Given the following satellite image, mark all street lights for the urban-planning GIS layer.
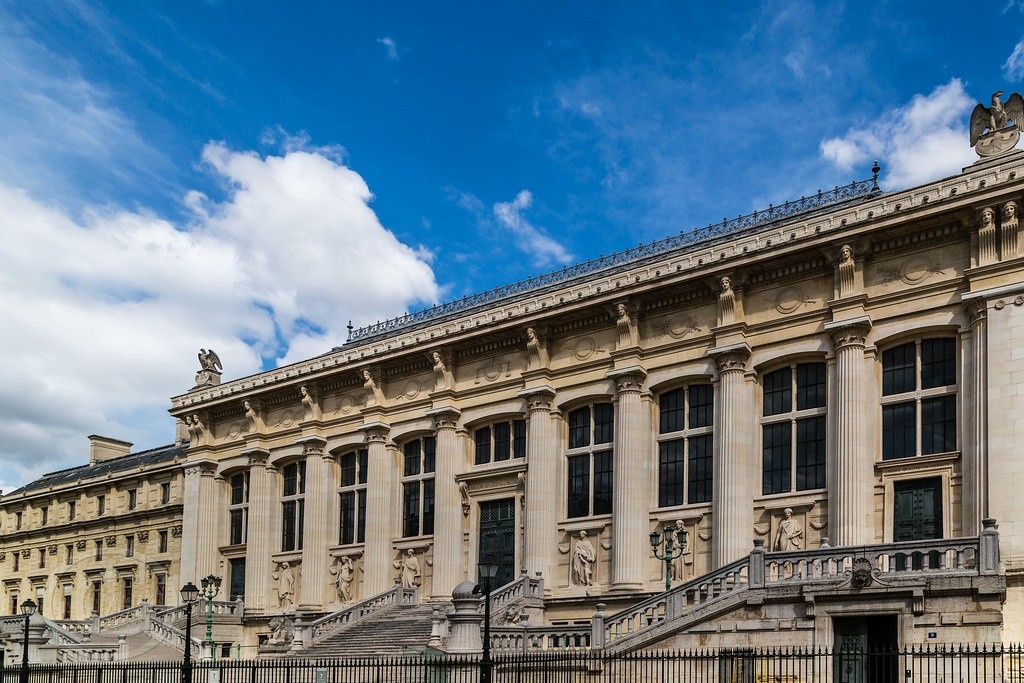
[179,581,199,683]
[648,525,688,592]
[19,599,38,683]
[471,553,500,683]
[201,574,222,641]
